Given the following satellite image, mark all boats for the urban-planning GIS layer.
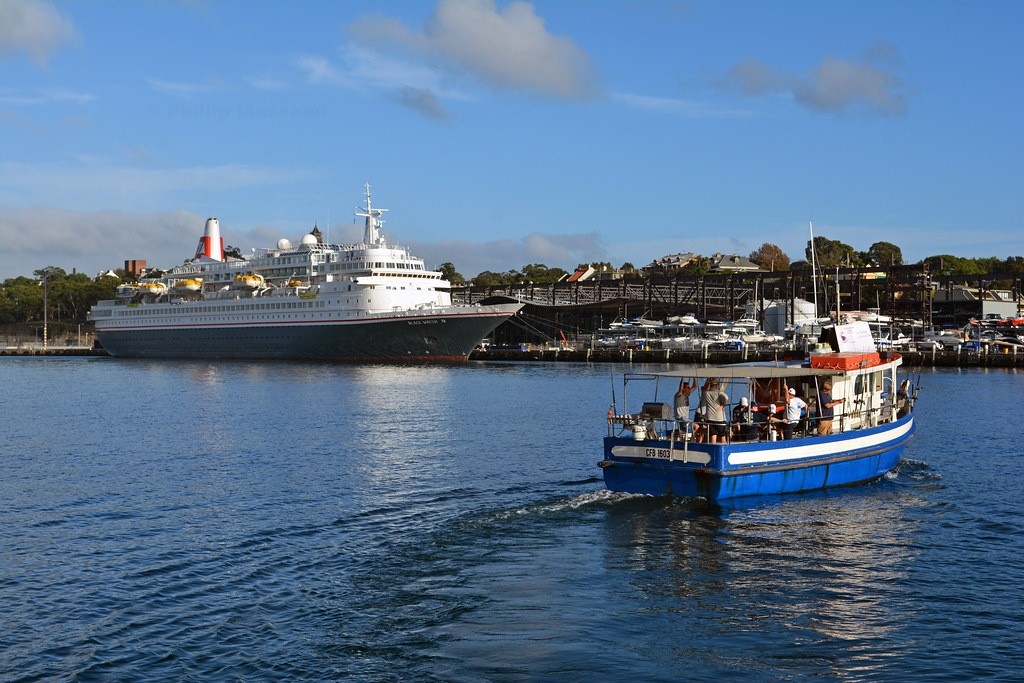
[600,318,919,494]
[519,308,1024,358]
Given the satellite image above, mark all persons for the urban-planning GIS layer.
[816,380,846,436]
[885,382,908,423]
[694,377,729,443]
[740,401,791,442]
[733,397,748,423]
[783,385,809,440]
[673,377,698,434]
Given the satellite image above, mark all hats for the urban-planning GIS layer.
[768,404,776,413]
[788,388,796,395]
[740,397,749,407]
[751,401,758,406]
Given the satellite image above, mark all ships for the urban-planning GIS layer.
[86,184,525,370]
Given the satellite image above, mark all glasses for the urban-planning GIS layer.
[686,387,690,389]
